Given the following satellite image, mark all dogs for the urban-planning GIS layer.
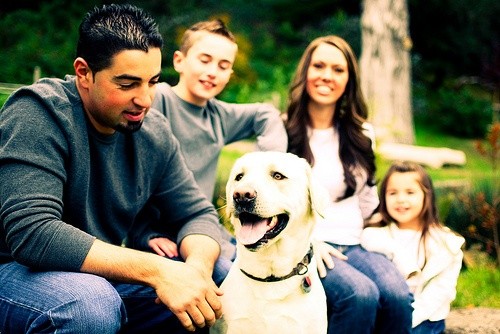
[207,151,328,334]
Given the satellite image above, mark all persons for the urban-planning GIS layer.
[274,34,415,334]
[123,16,288,262]
[360,159,465,334]
[0,3,235,334]
